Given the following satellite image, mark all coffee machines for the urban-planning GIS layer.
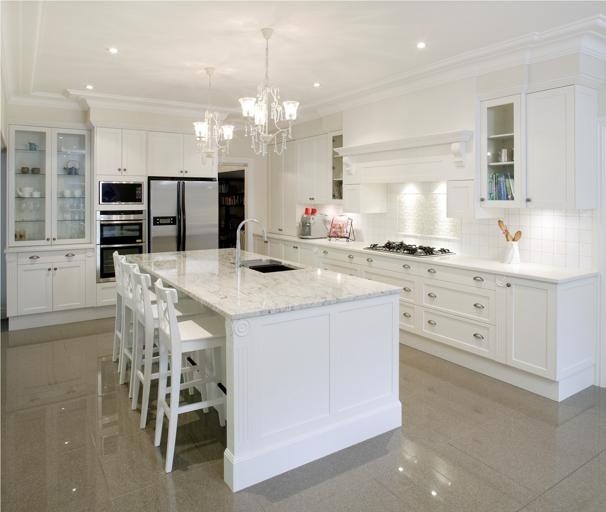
[298,206,329,238]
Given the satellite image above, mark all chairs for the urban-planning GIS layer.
[113,250,226,473]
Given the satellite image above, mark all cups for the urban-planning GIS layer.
[503,240,520,264]
[501,148,508,162]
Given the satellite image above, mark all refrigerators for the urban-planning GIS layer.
[148,175,219,255]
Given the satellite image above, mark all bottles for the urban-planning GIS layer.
[15,136,85,240]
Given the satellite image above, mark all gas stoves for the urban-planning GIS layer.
[364,240,455,258]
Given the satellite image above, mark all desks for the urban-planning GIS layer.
[125,247,405,494]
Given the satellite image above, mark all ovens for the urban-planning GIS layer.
[95,173,149,284]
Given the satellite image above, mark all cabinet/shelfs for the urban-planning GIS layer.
[267,142,297,234]
[297,135,328,204]
[96,127,147,176]
[253,236,282,261]
[146,130,213,177]
[328,130,343,203]
[7,124,91,247]
[16,259,85,315]
[473,88,525,209]
[525,85,598,211]
[284,240,319,270]
[505,277,599,382]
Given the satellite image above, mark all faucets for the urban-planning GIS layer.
[236,218,268,267]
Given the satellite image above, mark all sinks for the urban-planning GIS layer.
[249,263,305,274]
[230,258,282,267]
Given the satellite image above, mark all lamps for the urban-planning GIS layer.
[238,27,299,158]
[191,66,234,165]
